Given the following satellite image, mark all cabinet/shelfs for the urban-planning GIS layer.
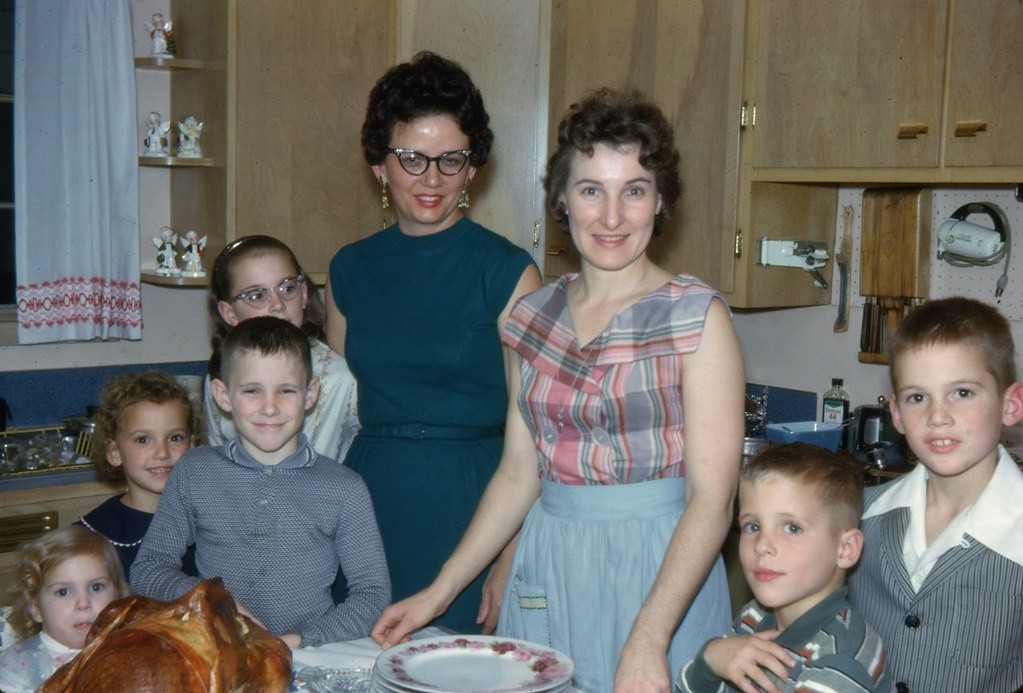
[0,479,131,608]
[542,0,1023,310]
[133,0,398,287]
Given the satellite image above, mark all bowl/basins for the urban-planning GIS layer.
[765,422,843,455]
[740,436,779,471]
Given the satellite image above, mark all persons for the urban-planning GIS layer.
[178,116,204,154]
[145,112,168,153]
[368,80,745,693]
[204,234,363,464]
[325,50,546,637]
[734,296,1023,693]
[150,226,179,269]
[151,13,169,54]
[130,315,392,650]
[180,230,207,273]
[676,440,892,693]
[72,368,202,584]
[0,524,124,693]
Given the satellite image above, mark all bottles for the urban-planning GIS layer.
[822,378,850,451]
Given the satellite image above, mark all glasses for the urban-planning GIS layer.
[226,267,304,309]
[384,146,472,176]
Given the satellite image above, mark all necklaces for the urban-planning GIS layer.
[554,258,653,431]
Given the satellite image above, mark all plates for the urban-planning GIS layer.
[369,634,576,693]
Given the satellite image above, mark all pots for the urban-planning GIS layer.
[62,404,98,436]
